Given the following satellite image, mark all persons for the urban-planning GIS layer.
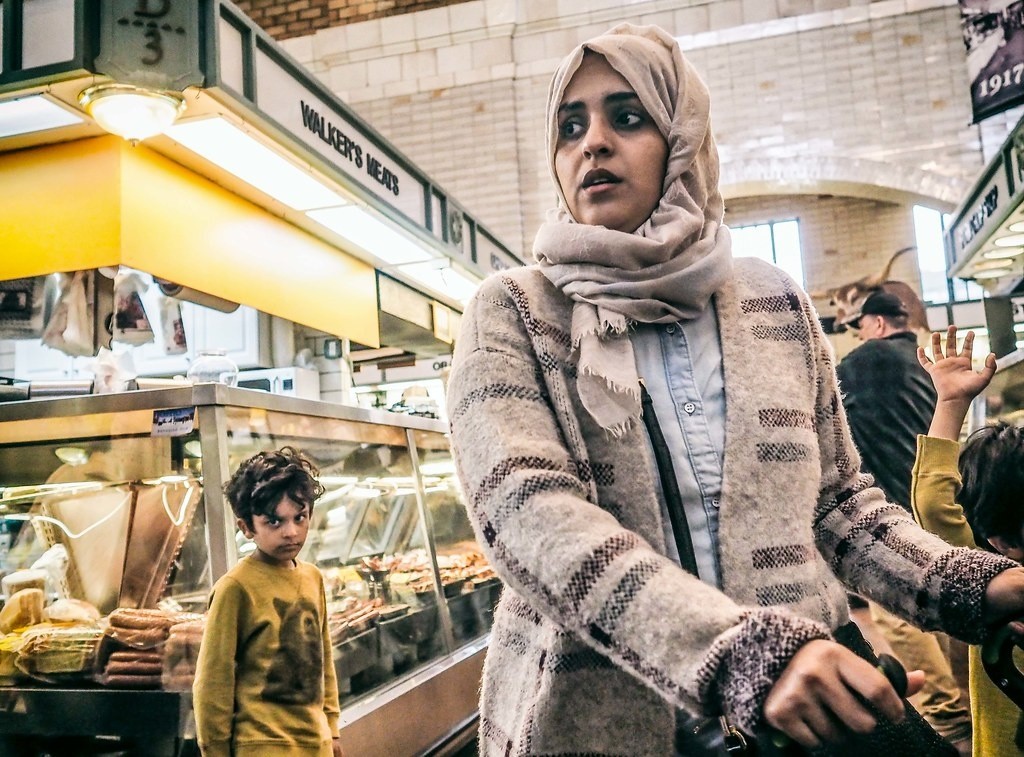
[823,291,972,757]
[192,448,342,757]
[911,326,1024,755]
[447,23,1024,757]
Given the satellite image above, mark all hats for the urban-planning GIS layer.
[840,292,910,329]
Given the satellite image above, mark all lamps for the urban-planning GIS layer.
[79,84,186,147]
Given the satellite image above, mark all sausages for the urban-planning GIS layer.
[327,597,381,638]
[101,607,208,690]
